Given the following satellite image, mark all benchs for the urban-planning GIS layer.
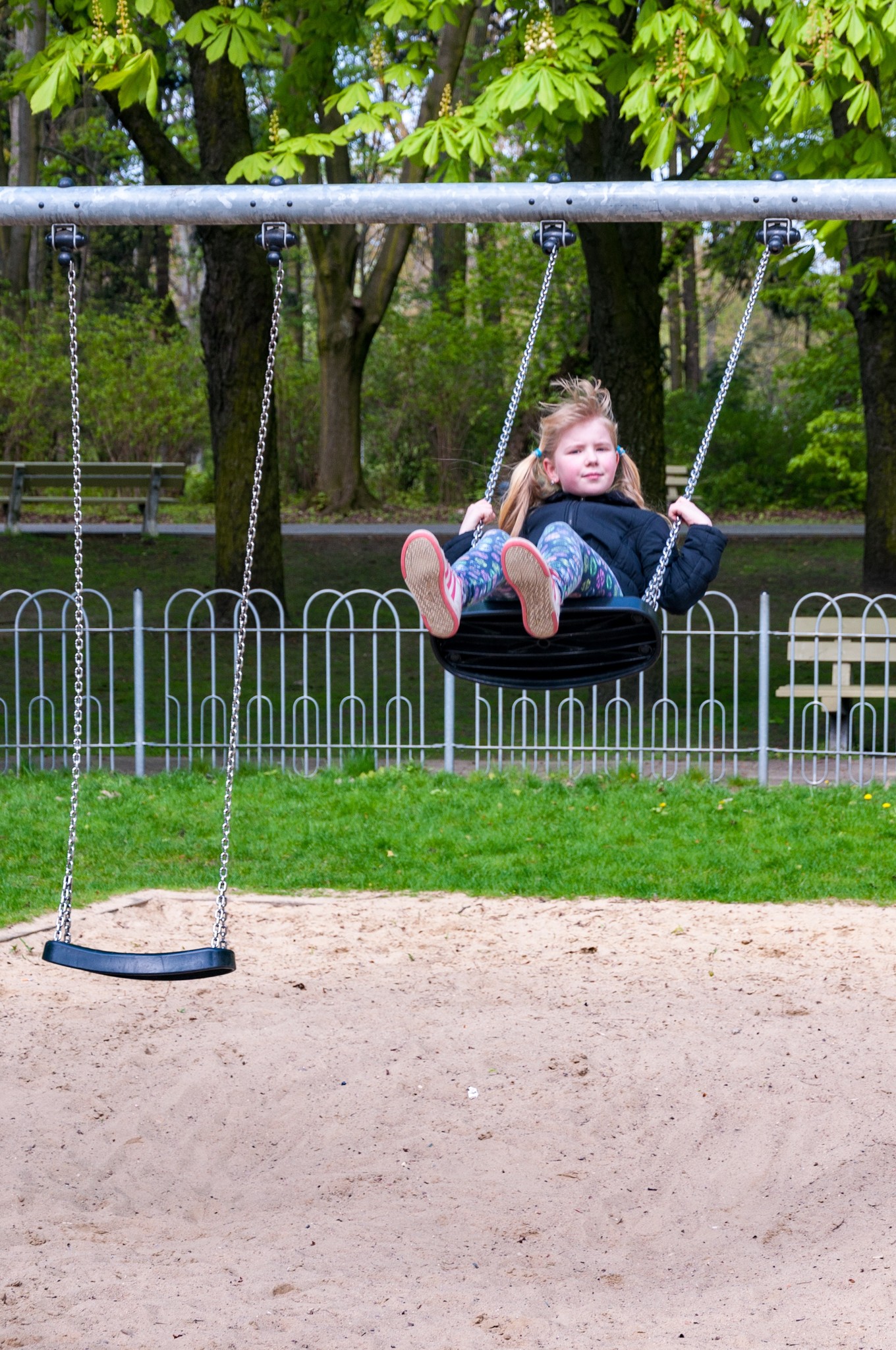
[0,461,186,543]
[776,616,896,752]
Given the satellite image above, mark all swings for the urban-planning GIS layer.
[430,244,770,690]
[40,257,285,981]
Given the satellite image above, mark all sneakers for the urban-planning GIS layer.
[402,528,464,638]
[501,537,561,639]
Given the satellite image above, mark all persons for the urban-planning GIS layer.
[400,377,727,639]
